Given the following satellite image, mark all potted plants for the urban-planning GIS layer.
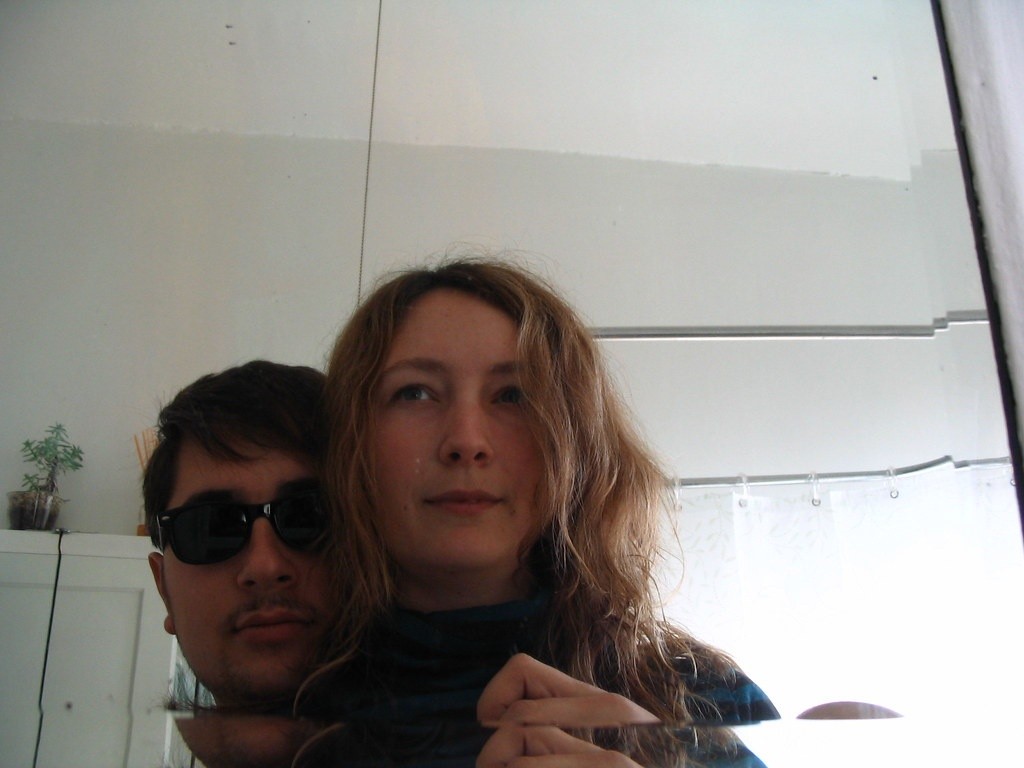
[4,422,85,530]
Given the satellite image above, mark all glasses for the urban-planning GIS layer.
[156,495,337,565]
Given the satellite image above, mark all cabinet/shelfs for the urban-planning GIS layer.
[0,529,179,768]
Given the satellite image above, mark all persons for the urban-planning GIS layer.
[143,258,776,723]
[177,715,764,768]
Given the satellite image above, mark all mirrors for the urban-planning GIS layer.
[0,0,1024,768]
